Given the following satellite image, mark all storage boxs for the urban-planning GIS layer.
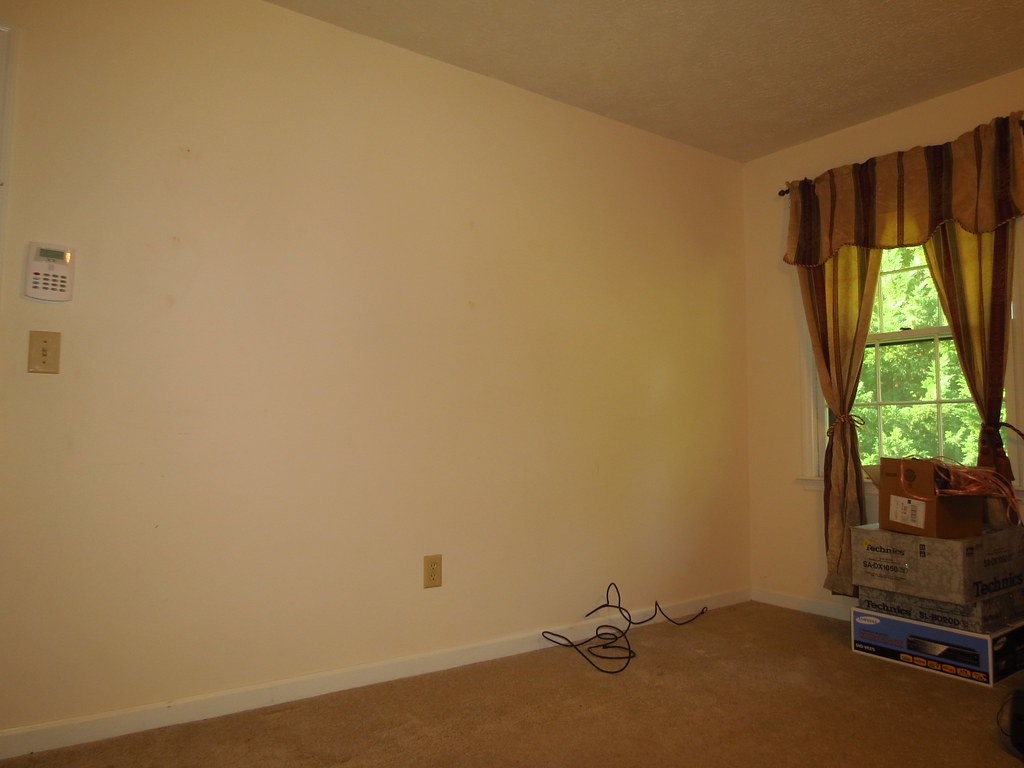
[850,458,1024,688]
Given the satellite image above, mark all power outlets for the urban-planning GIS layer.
[423,554,442,588]
